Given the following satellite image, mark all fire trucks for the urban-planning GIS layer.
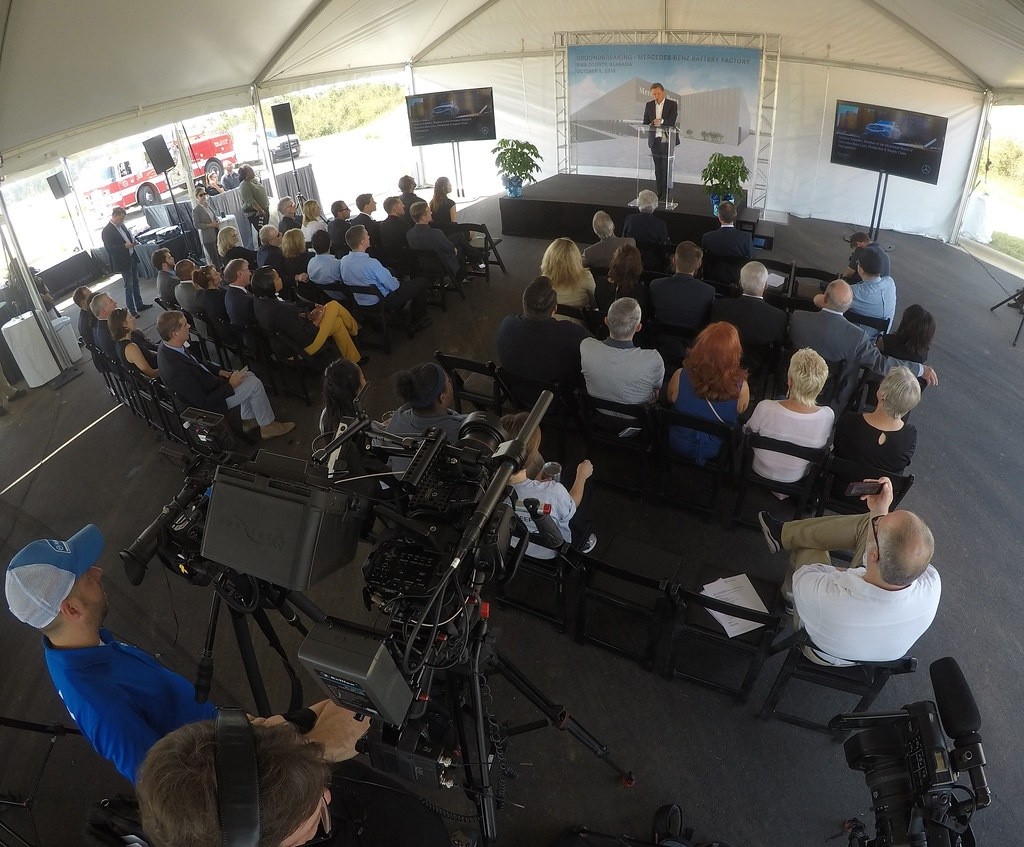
[80,127,240,223]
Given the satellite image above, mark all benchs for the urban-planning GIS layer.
[0,250,104,306]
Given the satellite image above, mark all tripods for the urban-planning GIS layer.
[396,600,635,847]
[194,565,327,721]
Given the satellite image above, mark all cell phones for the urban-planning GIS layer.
[845,482,884,497]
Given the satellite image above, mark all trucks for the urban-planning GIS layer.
[228,122,301,166]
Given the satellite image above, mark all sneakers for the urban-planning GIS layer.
[758,511,785,554]
[784,595,794,614]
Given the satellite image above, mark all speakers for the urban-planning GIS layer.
[46,171,71,200]
[271,102,295,137]
[143,134,175,175]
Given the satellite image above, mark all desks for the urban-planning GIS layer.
[197,214,244,266]
[133,229,199,279]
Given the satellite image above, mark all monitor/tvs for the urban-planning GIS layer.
[831,99,948,186]
[405,87,496,146]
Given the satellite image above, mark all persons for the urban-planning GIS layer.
[4,524,478,846]
[759,477,941,668]
[541,239,596,326]
[830,367,921,500]
[789,279,938,386]
[497,276,595,413]
[385,363,467,483]
[843,232,890,279]
[221,160,241,190]
[498,415,598,559]
[318,360,390,433]
[622,190,669,264]
[666,321,749,462]
[700,202,754,298]
[741,348,836,501]
[581,211,636,270]
[33,275,62,318]
[877,304,935,363]
[643,83,677,199]
[0,363,26,415]
[595,243,655,321]
[814,251,896,336]
[73,174,484,439]
[580,297,665,436]
[207,172,224,195]
[649,241,716,330]
[712,261,788,370]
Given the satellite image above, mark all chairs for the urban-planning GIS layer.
[78,221,932,745]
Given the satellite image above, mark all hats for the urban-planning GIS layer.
[6,523,104,628]
[29,267,38,274]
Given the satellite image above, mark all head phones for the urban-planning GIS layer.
[214,704,262,847]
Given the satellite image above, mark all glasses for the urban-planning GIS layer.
[871,514,886,562]
[271,230,280,241]
[198,193,206,197]
[292,788,334,847]
[340,207,350,213]
[287,202,296,208]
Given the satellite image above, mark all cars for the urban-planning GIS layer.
[865,119,902,141]
[432,100,459,119]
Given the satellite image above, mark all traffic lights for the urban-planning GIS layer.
[118,163,127,178]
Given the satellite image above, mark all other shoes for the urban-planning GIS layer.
[357,356,370,366]
[243,417,260,433]
[137,304,153,311]
[412,318,432,332]
[131,311,141,318]
[261,420,295,439]
[359,318,366,328]
[657,189,671,201]
[583,533,597,553]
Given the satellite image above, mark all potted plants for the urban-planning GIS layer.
[491,138,545,198]
[699,152,753,217]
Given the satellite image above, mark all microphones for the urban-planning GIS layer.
[460,390,555,552]
[930,657,991,808]
